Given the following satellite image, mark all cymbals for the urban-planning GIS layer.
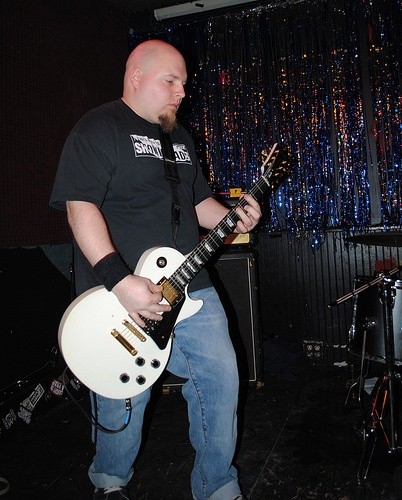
[343,231,402,247]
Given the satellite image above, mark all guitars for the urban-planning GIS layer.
[58,142,292,400]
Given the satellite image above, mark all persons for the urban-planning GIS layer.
[47,40,261,500]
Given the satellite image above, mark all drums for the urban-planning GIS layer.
[345,274,402,366]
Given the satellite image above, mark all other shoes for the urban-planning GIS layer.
[92,485,131,500]
